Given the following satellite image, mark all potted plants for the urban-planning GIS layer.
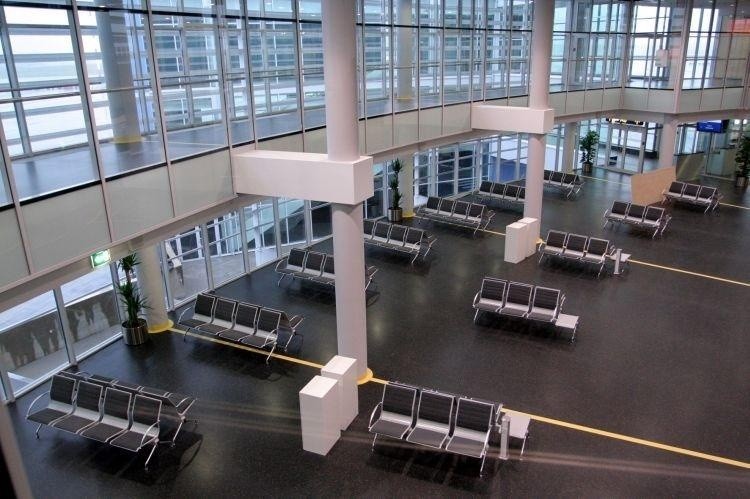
[578,130,599,173]
[387,158,402,221]
[116,253,149,346]
[734,136,750,188]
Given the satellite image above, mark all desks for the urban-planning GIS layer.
[610,253,632,272]
[556,312,581,342]
[497,411,532,461]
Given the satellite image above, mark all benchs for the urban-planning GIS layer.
[25,374,172,474]
[576,175,585,193]
[61,369,199,447]
[368,383,494,478]
[712,189,723,212]
[472,181,525,205]
[363,220,424,270]
[538,230,609,279]
[472,279,560,342]
[414,196,485,240]
[661,180,715,213]
[294,245,383,295]
[203,289,304,346]
[485,277,566,313]
[388,381,504,435]
[661,210,674,234]
[276,249,370,292]
[544,169,577,200]
[485,206,496,224]
[177,293,283,368]
[364,218,438,261]
[602,200,664,239]
